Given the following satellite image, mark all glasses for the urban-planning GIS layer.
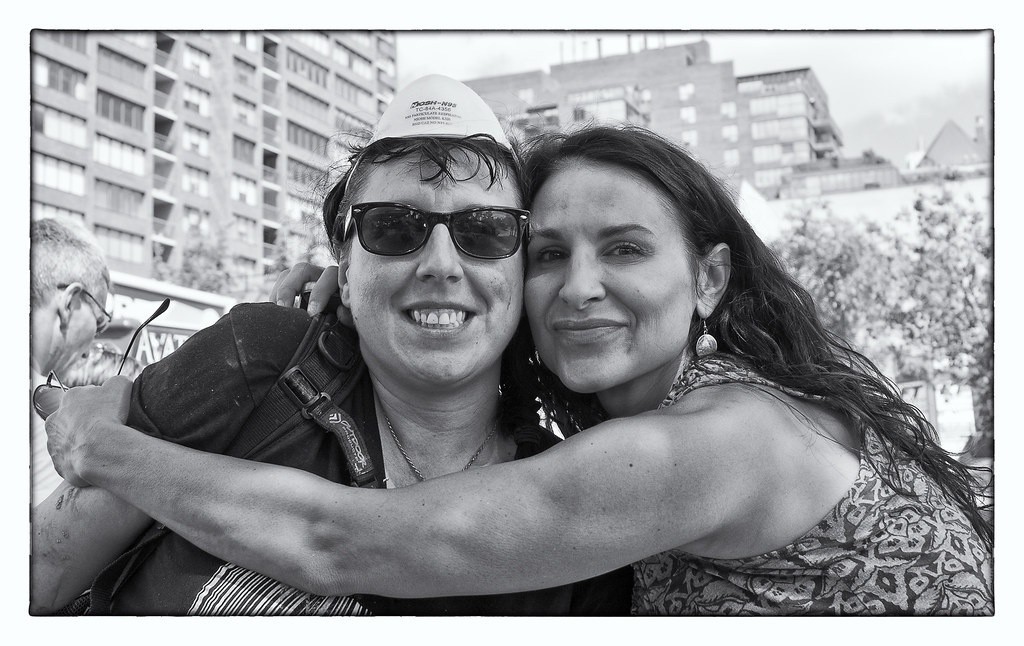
[33,298,170,422]
[57,284,114,335]
[342,201,531,260]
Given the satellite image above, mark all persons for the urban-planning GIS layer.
[44,123,995,617]
[29,76,635,617]
[30,218,144,511]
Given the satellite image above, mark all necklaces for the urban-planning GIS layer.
[376,394,497,482]
